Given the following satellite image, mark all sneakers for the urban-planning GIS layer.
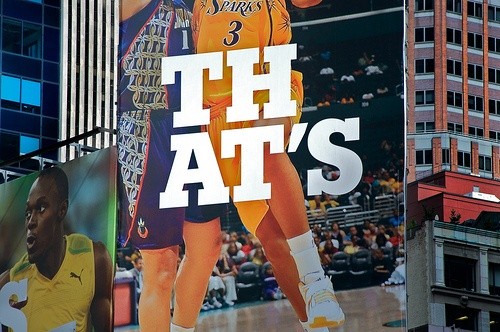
[297,270,345,328]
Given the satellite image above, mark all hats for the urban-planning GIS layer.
[386,241,393,248]
[235,241,242,250]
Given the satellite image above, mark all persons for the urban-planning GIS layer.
[192,0,346,332]
[300,141,404,212]
[118,219,406,311]
[0,165,115,332]
[117,0,222,332]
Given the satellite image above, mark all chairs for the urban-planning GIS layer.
[212,6,405,305]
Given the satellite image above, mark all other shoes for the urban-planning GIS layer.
[201,304,208,310]
[272,293,278,300]
[225,300,234,306]
[209,297,222,308]
[205,302,214,309]
